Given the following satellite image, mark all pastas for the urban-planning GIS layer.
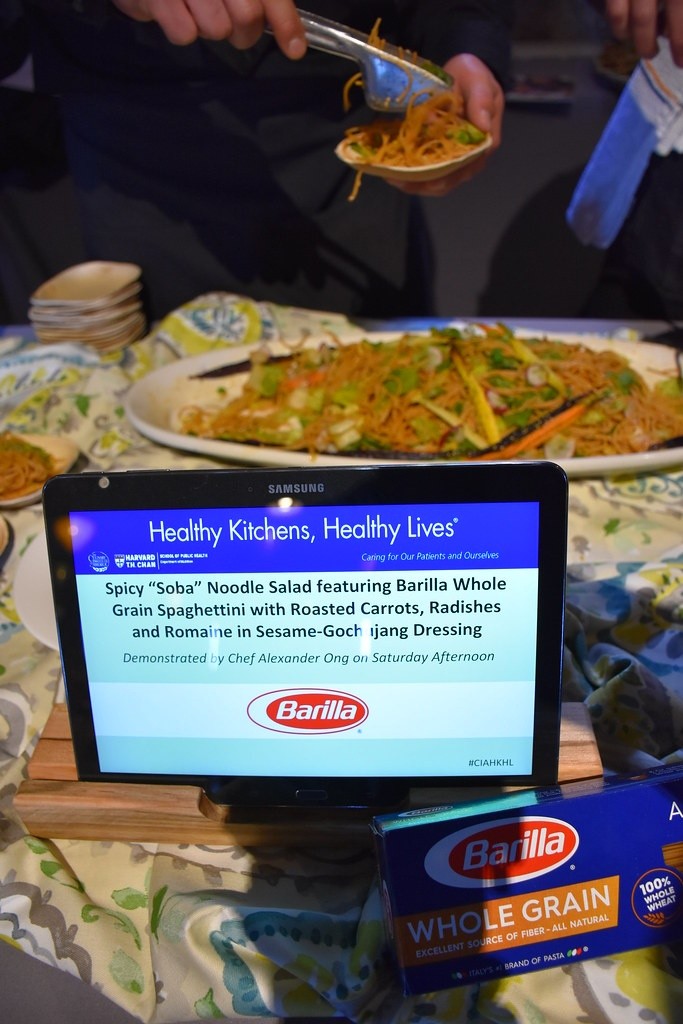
[344,17,485,200]
[0,429,61,500]
[176,333,683,456]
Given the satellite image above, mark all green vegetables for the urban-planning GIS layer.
[221,326,683,548]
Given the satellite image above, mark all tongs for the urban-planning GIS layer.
[262,8,454,114]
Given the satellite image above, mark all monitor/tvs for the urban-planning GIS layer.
[40,459,570,813]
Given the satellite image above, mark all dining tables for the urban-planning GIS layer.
[0,318,683,1024]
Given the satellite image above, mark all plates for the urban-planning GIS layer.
[27,259,148,355]
[335,131,494,183]
[0,433,81,510]
[13,528,60,653]
[124,327,683,479]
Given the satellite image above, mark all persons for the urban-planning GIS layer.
[34,0,508,317]
[580,0,683,319]
